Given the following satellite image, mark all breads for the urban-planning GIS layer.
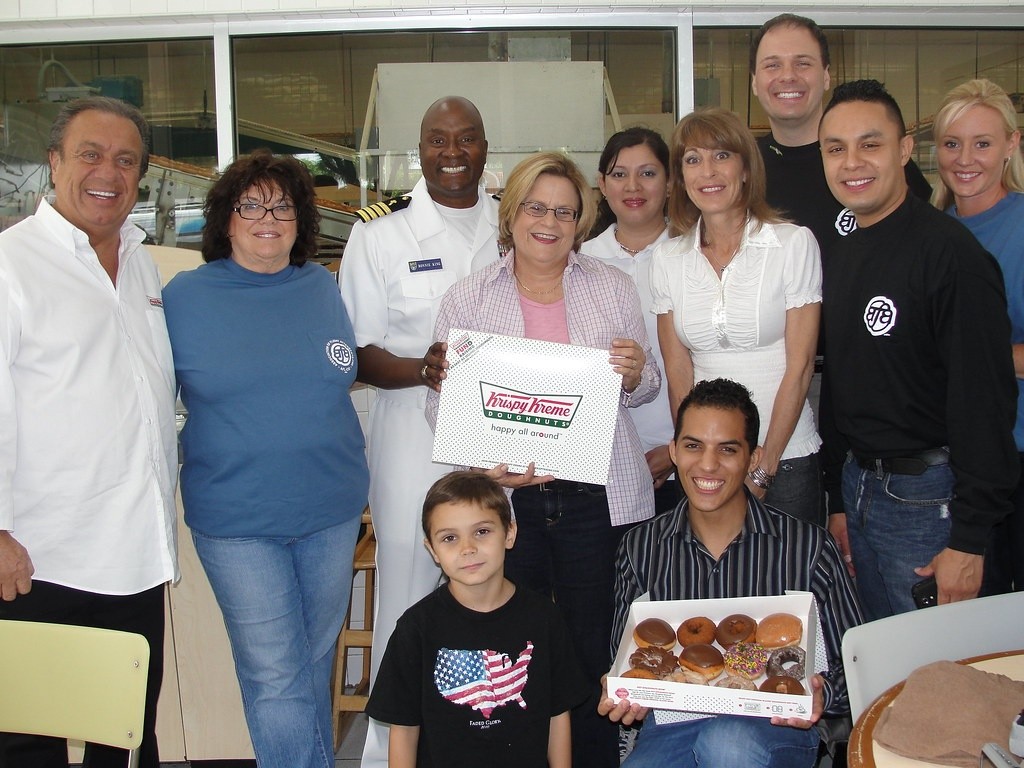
[678,643,724,682]
[755,613,803,652]
[633,618,676,649]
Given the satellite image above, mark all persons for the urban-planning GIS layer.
[648,108,826,527]
[596,378,864,768]
[160,151,370,768]
[336,95,512,768]
[750,13,933,241]
[425,151,661,768]
[816,77,1020,625]
[364,471,595,768]
[929,77,1024,472]
[0,96,184,768]
[576,125,672,516]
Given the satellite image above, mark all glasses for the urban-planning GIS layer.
[231,204,298,221]
[520,201,579,222]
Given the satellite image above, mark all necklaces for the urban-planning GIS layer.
[705,233,738,273]
[513,271,565,295]
[614,228,639,253]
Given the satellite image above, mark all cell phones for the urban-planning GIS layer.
[912,576,938,608]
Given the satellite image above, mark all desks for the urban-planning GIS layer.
[331,526,376,755]
[846,648,1024,768]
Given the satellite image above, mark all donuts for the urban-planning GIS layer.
[661,669,807,695]
[723,642,768,680]
[715,613,757,650]
[677,617,716,648]
[620,646,678,680]
[765,645,805,680]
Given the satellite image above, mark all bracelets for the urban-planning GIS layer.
[748,467,776,490]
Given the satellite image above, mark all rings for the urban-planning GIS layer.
[632,360,637,368]
[421,365,428,378]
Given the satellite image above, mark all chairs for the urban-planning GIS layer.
[0,620,153,768]
[839,589,1024,726]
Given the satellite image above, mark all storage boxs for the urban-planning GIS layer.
[427,328,627,488]
[604,590,830,721]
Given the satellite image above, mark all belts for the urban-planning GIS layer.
[858,447,950,475]
[525,480,604,491]
